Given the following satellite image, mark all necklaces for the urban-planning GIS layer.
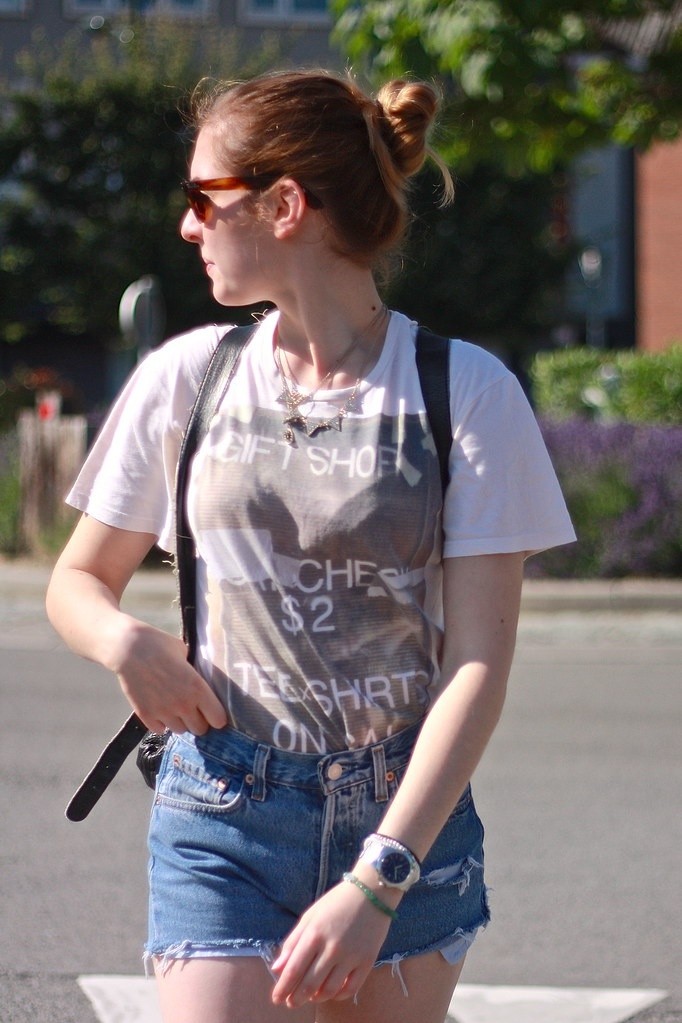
[275,305,389,445]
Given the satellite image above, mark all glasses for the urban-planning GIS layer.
[181,175,327,224]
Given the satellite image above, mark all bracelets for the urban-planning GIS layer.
[342,873,399,920]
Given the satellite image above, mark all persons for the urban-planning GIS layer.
[46,71,578,1023]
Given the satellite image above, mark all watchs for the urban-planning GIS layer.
[359,835,419,890]
